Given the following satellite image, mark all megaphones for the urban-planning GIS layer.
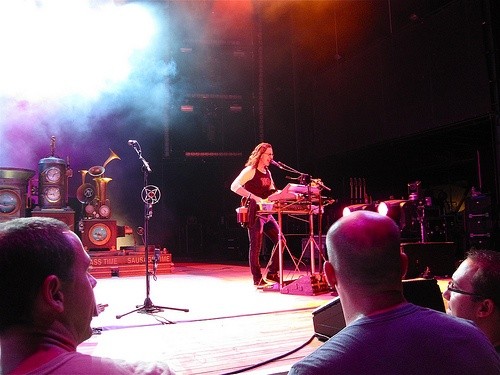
[76,148,121,206]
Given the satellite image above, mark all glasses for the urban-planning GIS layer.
[264,153,275,156]
[447,281,489,298]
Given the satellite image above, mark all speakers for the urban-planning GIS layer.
[313,299,346,342]
[401,278,446,314]
[434,244,464,274]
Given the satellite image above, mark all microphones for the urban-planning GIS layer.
[270,159,283,170]
[128,140,137,145]
[277,161,286,167]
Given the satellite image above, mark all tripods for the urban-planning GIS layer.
[116,146,189,318]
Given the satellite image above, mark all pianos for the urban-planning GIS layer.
[255,203,324,215]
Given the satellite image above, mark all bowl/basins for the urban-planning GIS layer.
[259,203,273,210]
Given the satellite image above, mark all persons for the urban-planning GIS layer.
[231,143,286,288]
[444,248,500,356]
[288,210,500,375]
[0,217,172,375]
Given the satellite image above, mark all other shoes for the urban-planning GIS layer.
[267,273,280,283]
[254,278,268,285]
